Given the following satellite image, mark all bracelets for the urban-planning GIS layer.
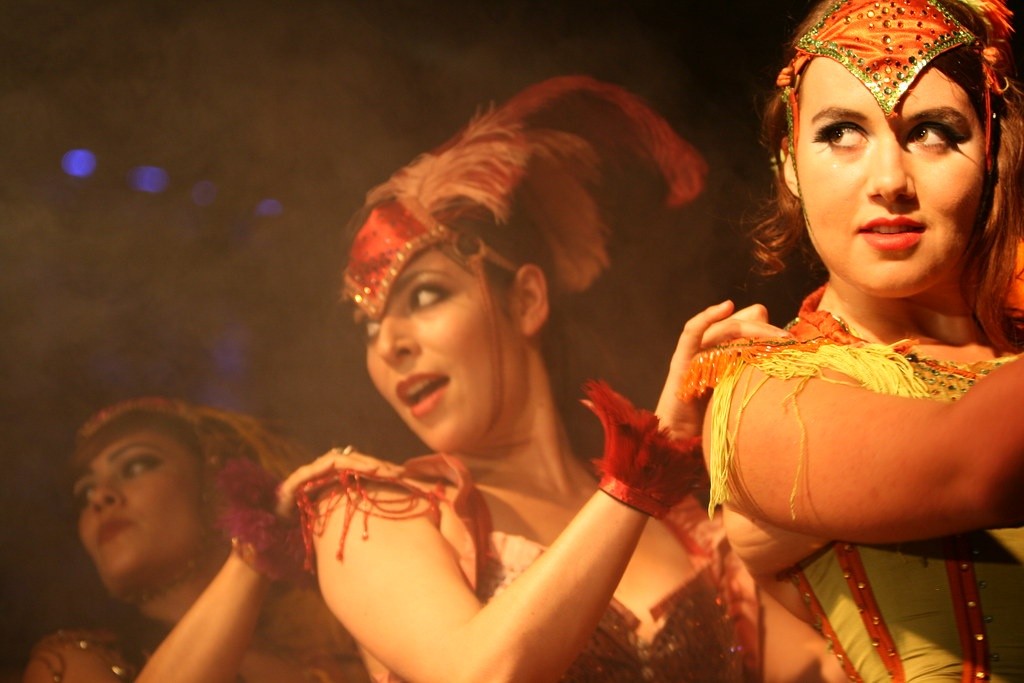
[580,380,703,520]
[212,451,305,579]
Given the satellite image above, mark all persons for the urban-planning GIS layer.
[298,76,794,683]
[681,1,1024,683]
[24,397,395,683]
